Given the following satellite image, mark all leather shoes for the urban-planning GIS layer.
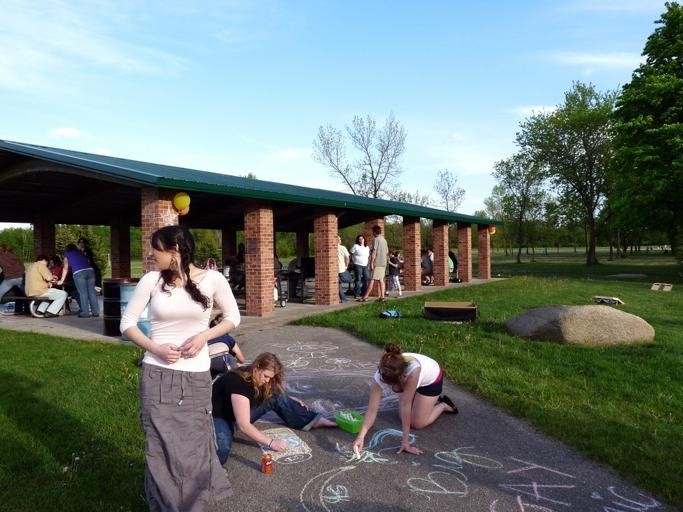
[437,396,457,414]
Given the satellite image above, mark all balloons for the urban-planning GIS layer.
[174,192,193,215]
[489,226,497,237]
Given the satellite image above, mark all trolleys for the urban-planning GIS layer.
[275,270,290,308]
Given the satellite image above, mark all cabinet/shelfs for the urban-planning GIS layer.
[277,254,316,305]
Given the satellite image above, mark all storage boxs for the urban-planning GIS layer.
[421,299,477,323]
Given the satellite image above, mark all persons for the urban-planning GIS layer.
[335,224,457,303]
[209,314,244,378]
[351,343,458,457]
[228,239,283,290]
[0,234,103,318]
[120,226,241,511]
[208,351,340,469]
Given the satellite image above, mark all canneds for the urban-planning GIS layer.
[261,454,273,474]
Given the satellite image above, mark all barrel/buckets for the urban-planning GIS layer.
[102,275,141,337]
[119,282,151,341]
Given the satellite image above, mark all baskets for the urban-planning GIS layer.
[334,410,363,434]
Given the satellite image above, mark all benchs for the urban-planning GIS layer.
[0,295,49,318]
